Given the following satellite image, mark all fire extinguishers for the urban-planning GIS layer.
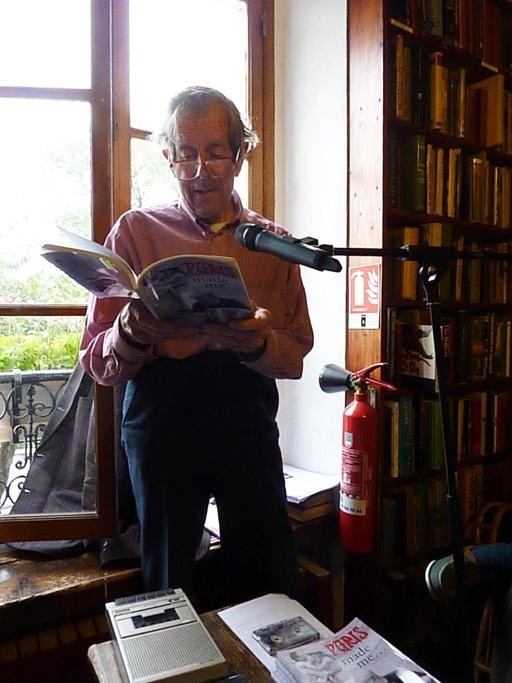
[319,363,397,553]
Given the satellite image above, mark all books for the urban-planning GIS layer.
[270,618,441,683]
[42,227,257,331]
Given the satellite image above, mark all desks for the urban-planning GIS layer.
[86,591,335,683]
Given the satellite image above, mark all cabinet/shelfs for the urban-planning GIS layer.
[346,1,512,683]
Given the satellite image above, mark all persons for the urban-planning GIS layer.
[420,545,512,603]
[78,84,315,611]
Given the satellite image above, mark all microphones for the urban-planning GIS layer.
[235,223,342,272]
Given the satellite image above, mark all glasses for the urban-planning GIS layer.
[167,144,240,181]
